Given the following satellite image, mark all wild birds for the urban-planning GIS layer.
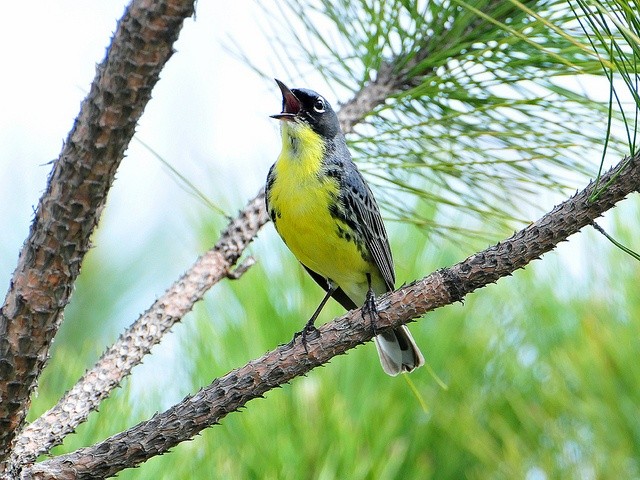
[264,78,425,377]
